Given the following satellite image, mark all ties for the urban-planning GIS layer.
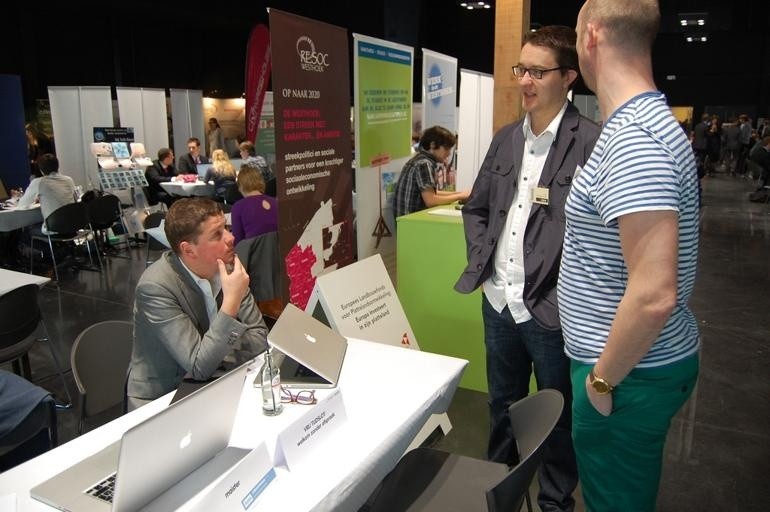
[195,158,198,164]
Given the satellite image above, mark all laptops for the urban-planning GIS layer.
[27,359,249,512]
[249,301,349,392]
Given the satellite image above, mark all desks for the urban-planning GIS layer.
[0,333,471,512]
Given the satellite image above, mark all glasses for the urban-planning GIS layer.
[279,385,317,405]
[512,65,563,80]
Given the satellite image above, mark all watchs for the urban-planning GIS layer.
[588,365,617,400]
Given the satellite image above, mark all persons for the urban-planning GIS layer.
[128,194,271,416]
[453,25,604,512]
[393,125,472,226]
[677,107,770,202]
[554,0,702,510]
[437,161,457,189]
[141,115,278,243]
[411,132,420,155]
[9,152,78,275]
[24,123,54,174]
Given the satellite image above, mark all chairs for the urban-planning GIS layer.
[361,388,566,509]
[688,139,769,204]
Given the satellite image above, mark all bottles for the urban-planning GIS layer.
[259,352,283,416]
